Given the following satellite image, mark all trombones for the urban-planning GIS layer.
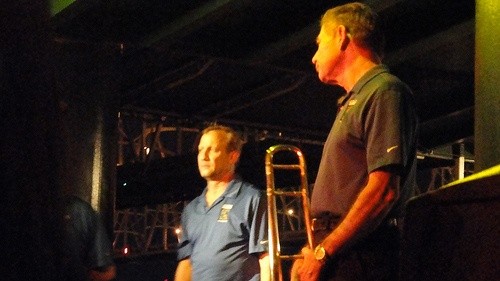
[263,143,318,281]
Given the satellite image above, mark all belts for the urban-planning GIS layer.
[310,218,399,233]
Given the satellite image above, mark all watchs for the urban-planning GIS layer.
[313,244,331,266]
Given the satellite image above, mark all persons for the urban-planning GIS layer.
[64,196,116,281]
[290,1,424,281]
[173,125,268,281]
